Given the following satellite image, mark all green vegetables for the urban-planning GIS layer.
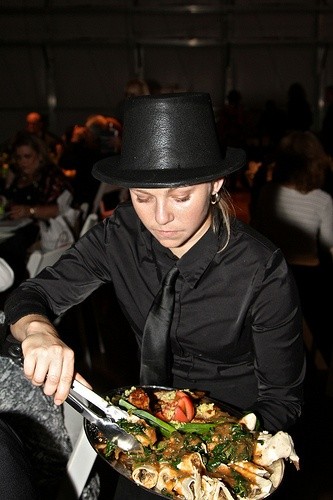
[91,386,265,499]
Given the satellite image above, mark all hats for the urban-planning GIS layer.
[92,92,248,188]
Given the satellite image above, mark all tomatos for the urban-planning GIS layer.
[158,391,194,423]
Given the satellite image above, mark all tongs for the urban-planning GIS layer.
[7,343,145,452]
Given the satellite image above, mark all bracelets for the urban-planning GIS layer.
[30,206,35,217]
[23,205,28,217]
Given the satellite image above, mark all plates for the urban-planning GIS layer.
[83,385,285,500]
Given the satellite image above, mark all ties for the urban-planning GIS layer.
[140,266,180,387]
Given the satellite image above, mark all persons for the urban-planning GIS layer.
[4,92,312,500]
[0,79,333,264]
